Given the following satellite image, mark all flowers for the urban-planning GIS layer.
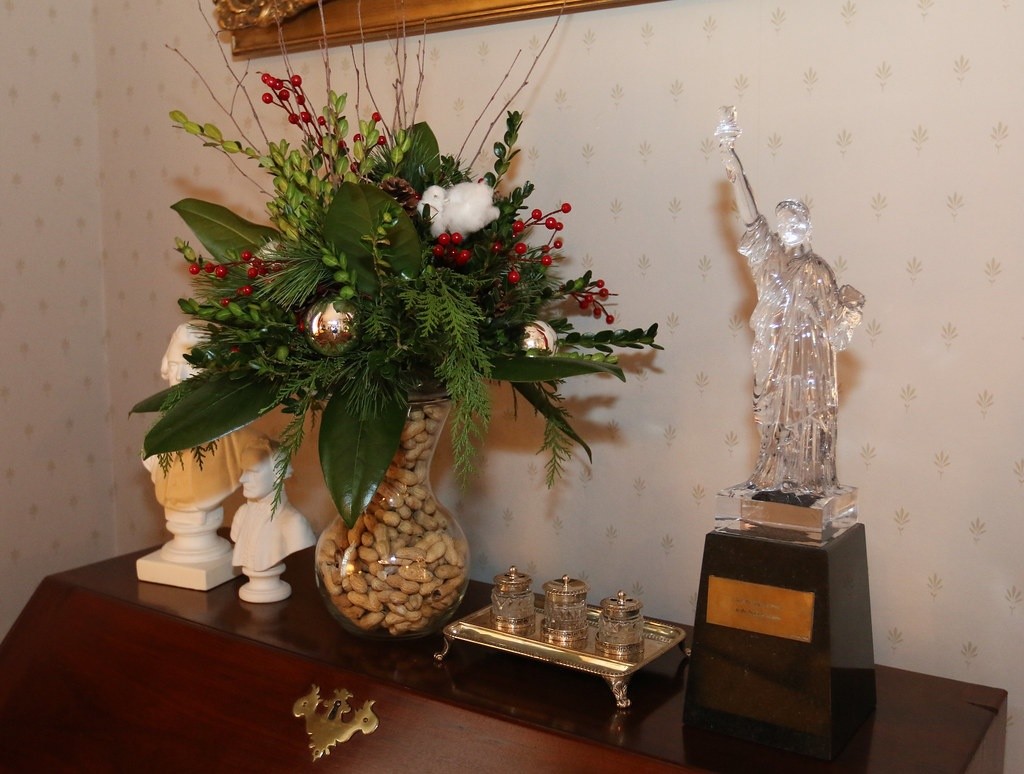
[127,0,664,533]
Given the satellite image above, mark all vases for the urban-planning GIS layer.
[314,386,473,646]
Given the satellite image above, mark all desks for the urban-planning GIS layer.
[0,526,1009,774]
